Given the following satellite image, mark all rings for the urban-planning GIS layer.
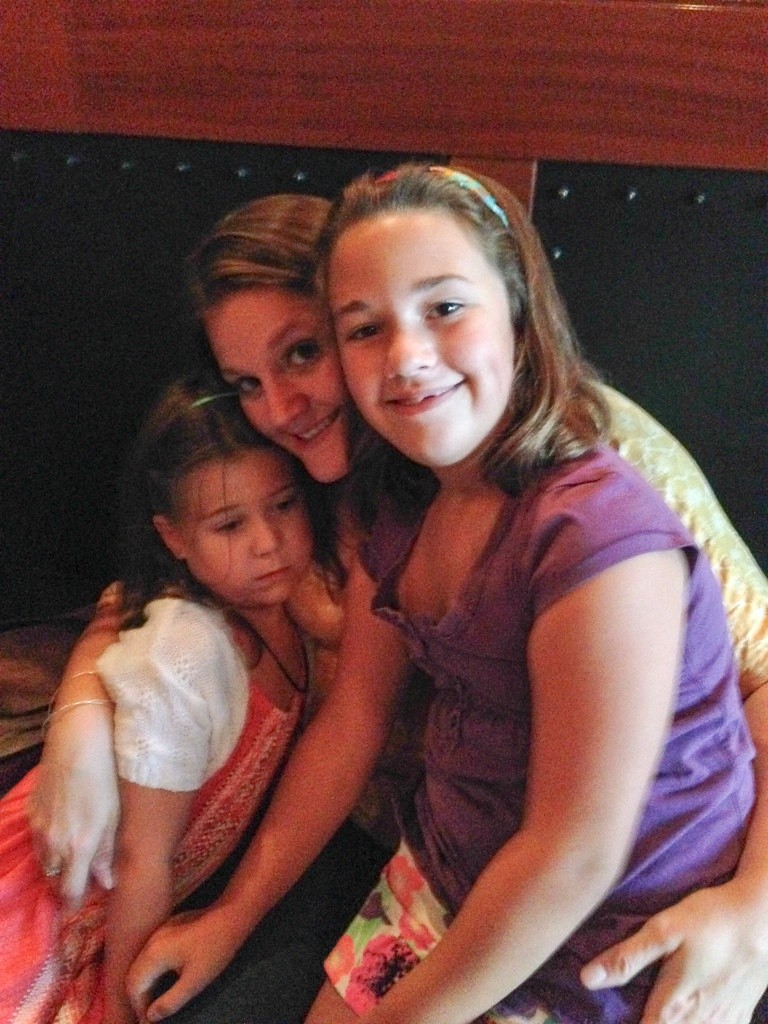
[43,866,61,878]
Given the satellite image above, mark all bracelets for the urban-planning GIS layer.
[41,698,114,741]
[46,671,100,715]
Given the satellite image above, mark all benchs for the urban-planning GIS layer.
[0,127,768,632]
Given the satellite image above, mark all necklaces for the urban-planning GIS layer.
[235,610,310,696]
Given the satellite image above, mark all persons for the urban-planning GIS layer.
[25,192,768,1024]
[0,364,343,1024]
[127,157,756,1024]
